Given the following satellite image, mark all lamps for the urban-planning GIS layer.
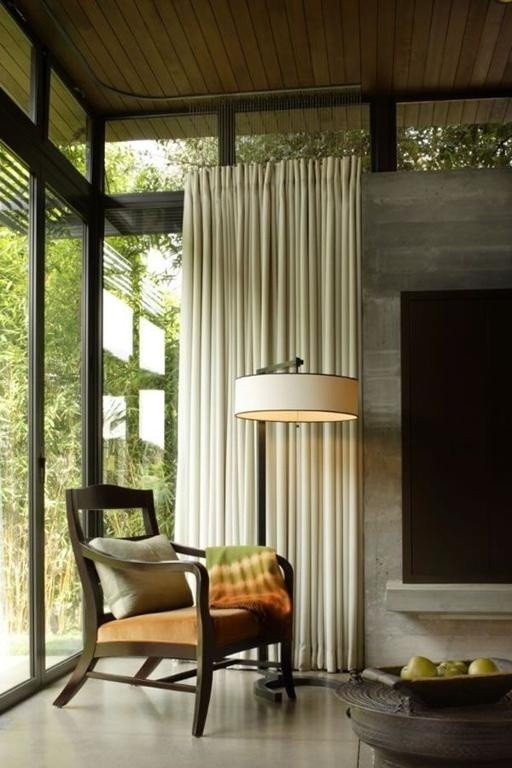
[234,358,360,705]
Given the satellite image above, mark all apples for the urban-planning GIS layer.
[400,657,499,680]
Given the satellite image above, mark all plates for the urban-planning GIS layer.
[359,656,511,709]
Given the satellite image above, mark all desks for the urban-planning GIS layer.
[331,657,510,767]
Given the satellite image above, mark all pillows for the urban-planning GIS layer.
[87,532,194,620]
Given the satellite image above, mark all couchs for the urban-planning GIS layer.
[50,484,296,737]
